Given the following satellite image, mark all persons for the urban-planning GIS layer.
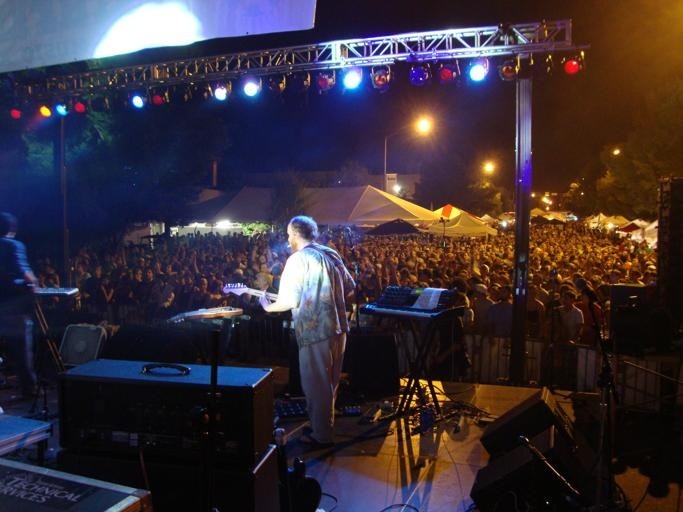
[358,225,658,392]
[266,244,280,287]
[260,263,269,272]
[258,215,357,446]
[0,211,39,394]
[251,242,267,271]
[41,226,247,315]
[251,272,278,321]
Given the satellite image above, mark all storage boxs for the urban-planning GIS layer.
[57,356,272,455]
[0,458,151,512]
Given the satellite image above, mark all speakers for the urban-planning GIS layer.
[606,280,658,356]
[660,176,683,353]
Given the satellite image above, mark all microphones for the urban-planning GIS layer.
[575,276,598,303]
[344,226,354,250]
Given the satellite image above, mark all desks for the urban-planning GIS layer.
[0,413,52,461]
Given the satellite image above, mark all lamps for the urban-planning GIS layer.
[467,57,489,81]
[407,63,431,86]
[436,62,458,81]
[9,106,23,120]
[149,87,169,106]
[546,48,587,84]
[343,67,362,89]
[55,101,69,116]
[292,71,310,90]
[91,97,109,112]
[175,84,191,104]
[315,70,335,91]
[515,52,536,78]
[40,104,54,118]
[498,56,516,82]
[74,96,87,113]
[214,80,232,101]
[370,69,394,89]
[266,73,285,91]
[198,84,213,103]
[128,90,147,111]
[244,77,262,97]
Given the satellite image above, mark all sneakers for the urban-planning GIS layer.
[295,431,336,449]
[23,386,49,400]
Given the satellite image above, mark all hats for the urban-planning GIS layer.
[474,283,487,293]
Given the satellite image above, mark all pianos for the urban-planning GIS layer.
[35,286,79,297]
[361,285,464,321]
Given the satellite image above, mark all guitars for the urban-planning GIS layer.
[167,305,243,323]
[222,283,281,302]
